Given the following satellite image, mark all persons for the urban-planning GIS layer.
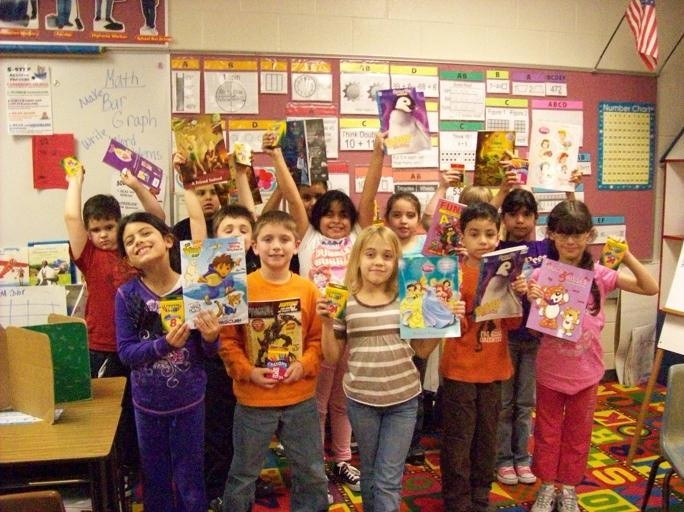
[61,126,583,511]
[528,200,662,512]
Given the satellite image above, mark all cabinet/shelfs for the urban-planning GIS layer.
[651,127,683,386]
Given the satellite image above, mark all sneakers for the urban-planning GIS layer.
[496,465,579,512]
[334,462,361,492]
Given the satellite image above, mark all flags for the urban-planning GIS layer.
[625,0,659,73]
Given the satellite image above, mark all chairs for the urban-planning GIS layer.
[0,487,67,511]
[640,359,683,511]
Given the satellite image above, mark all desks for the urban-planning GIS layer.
[0,374,129,511]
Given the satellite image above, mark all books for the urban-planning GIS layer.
[282,119,330,189]
[526,258,594,343]
[374,87,433,157]
[0,240,77,287]
[169,110,233,191]
